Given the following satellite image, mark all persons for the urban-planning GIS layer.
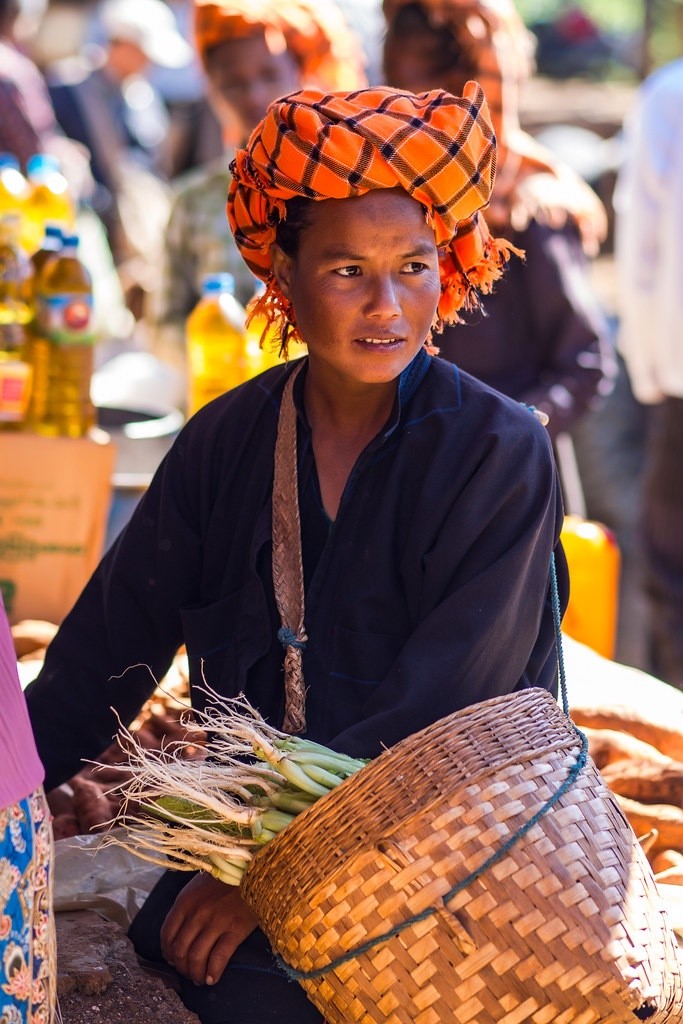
[1,591,66,1023]
[14,82,576,1023]
[1,0,682,697]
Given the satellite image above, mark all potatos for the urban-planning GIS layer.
[38,702,210,843]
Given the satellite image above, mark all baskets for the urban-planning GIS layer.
[238,685,683,1024]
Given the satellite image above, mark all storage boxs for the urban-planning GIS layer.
[0,421,118,628]
[239,687,683,1024]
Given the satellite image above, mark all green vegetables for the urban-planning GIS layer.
[60,659,377,888]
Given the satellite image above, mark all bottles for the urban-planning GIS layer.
[10,274,11,275]
[47,238,96,441]
[0,216,32,428]
[185,274,247,419]
[31,228,62,438]
[0,152,31,255]
[247,278,312,381]
[28,152,75,253]
